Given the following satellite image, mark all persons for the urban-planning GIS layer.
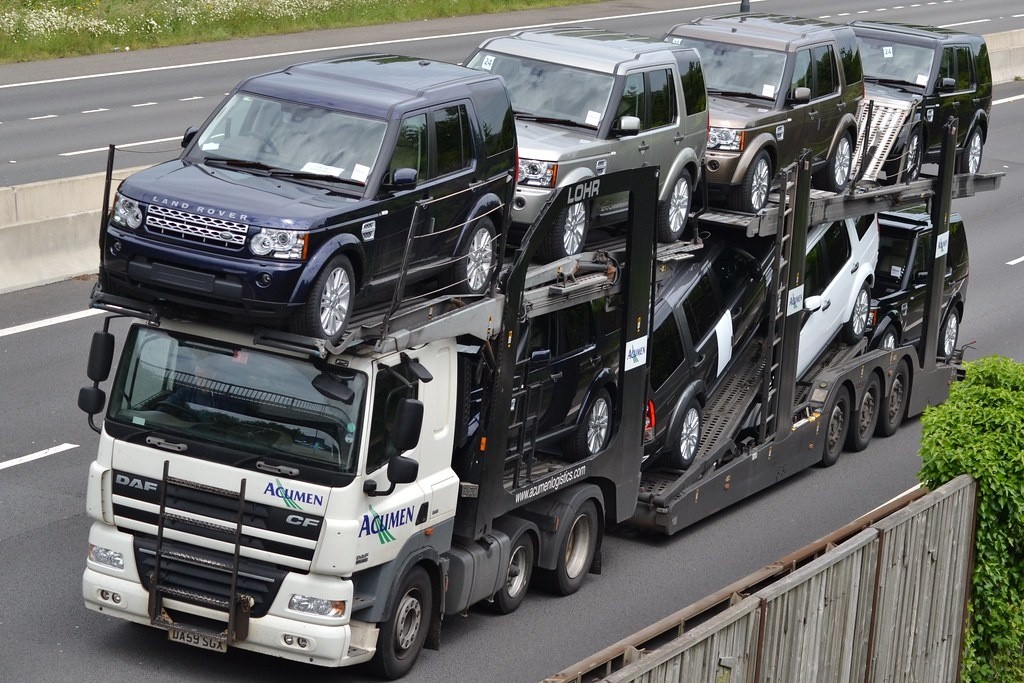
[160,365,231,415]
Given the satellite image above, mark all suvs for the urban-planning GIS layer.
[655,10,866,220]
[461,196,972,476]
[456,20,710,267]
[97,50,520,347]
[842,16,995,188]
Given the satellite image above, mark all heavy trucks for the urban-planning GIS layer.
[76,162,963,683]
